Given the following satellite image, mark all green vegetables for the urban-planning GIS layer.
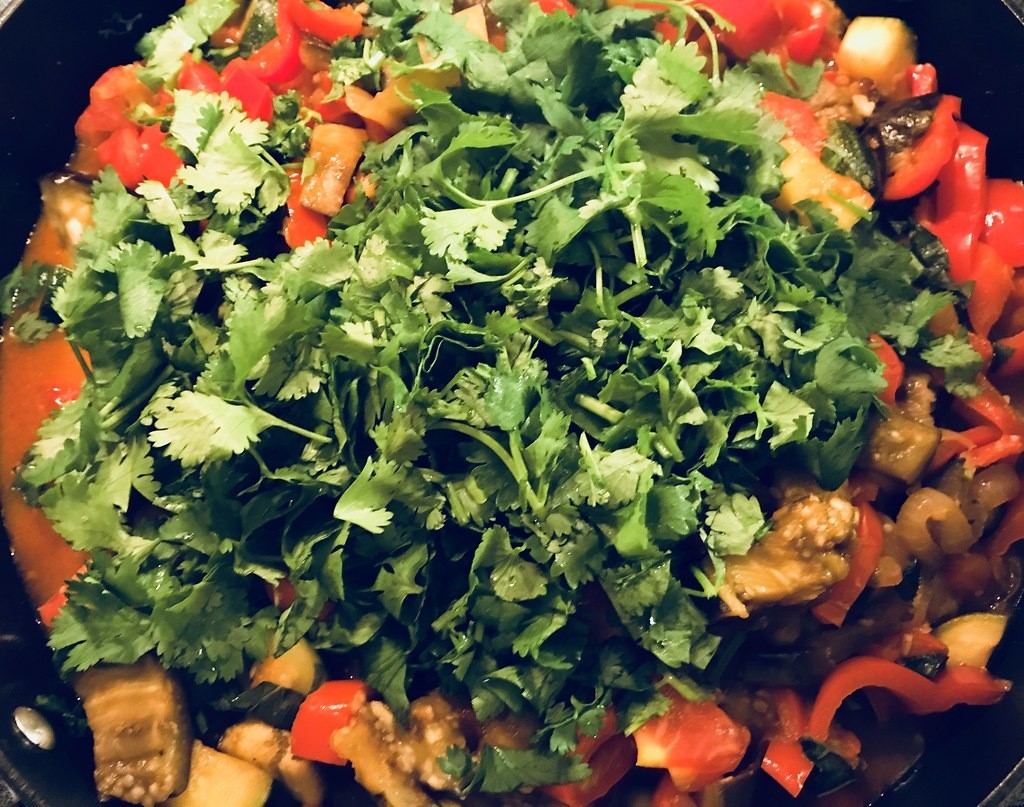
[0,0,984,799]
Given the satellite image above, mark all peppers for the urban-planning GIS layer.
[72,2,1024,807]
